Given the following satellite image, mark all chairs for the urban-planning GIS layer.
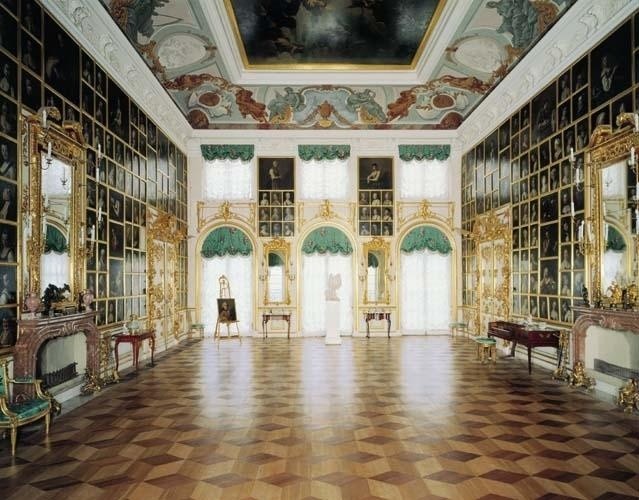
[0,356,53,455]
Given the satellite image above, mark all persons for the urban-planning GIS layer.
[367,163,384,189]
[259,191,294,237]
[360,192,393,235]
[460,70,587,323]
[0,0,187,346]
[269,160,281,190]
[221,303,234,320]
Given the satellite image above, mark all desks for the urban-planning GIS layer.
[489,316,564,378]
[363,311,391,340]
[261,313,291,341]
[112,329,156,375]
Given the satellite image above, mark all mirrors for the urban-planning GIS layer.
[12,105,89,321]
[362,234,390,306]
[262,235,291,306]
[583,108,639,313]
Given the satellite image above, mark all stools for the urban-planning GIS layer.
[472,335,497,361]
